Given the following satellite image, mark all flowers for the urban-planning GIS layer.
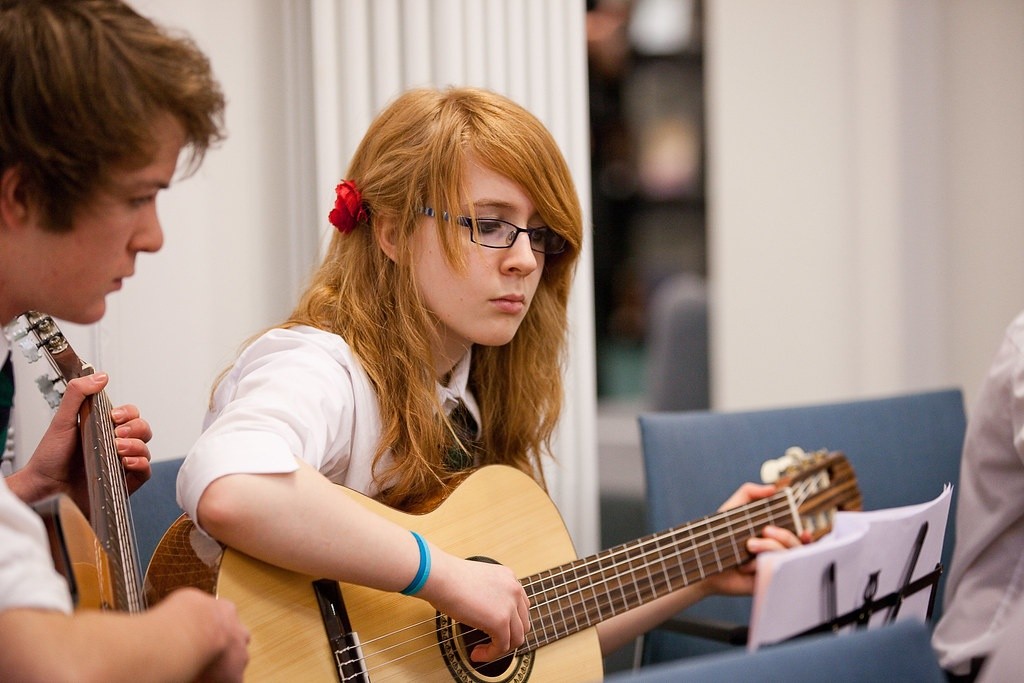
[327,177,369,234]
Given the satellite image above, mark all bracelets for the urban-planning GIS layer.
[399,531,431,595]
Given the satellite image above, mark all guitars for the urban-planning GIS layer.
[142,443,862,683]
[7,308,146,614]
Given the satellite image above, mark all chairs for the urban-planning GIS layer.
[632,388,966,671]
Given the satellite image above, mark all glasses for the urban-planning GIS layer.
[420,202,567,255]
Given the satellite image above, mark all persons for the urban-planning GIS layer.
[176,85,810,663]
[0,0,249,683]
[931,314,1024,683]
